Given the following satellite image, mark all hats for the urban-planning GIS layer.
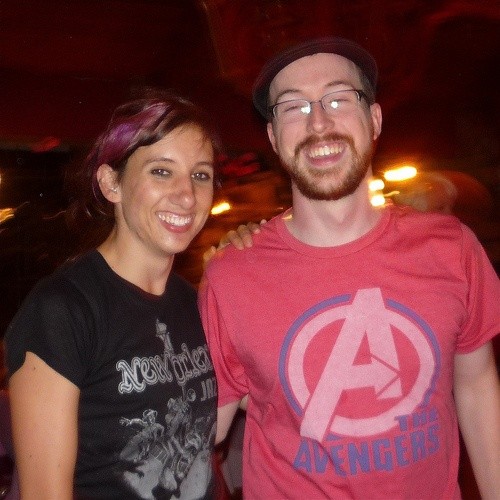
[253,35,379,123]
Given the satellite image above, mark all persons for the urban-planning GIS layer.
[8,95,267,500]
[196,39,498,500]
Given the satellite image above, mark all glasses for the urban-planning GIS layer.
[269,89,371,125]
